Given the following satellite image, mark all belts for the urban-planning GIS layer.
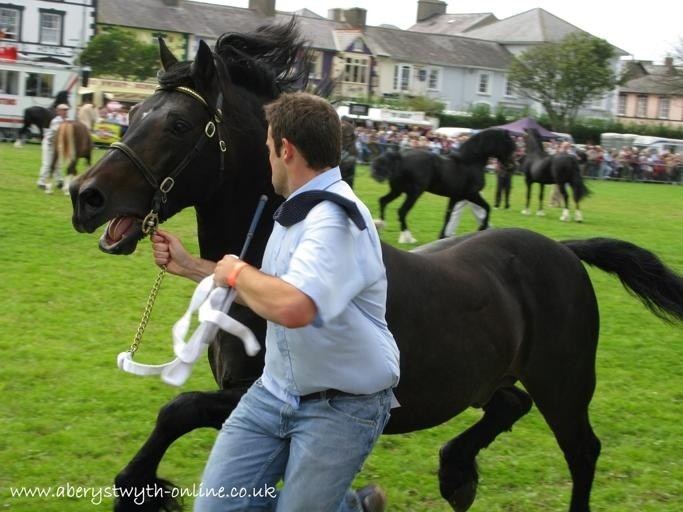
[295,388,366,405]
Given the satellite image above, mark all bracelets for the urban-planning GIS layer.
[229,262,249,288]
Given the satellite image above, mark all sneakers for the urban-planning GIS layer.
[355,483,387,512]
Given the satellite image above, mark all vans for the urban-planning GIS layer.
[549,132,574,144]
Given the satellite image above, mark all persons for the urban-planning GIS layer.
[354,123,683,185]
[151,90,402,512]
[95,107,129,127]
[444,191,486,236]
[37,103,69,193]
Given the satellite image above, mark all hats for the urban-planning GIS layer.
[55,103,69,112]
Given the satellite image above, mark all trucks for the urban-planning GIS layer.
[0,47,91,142]
[432,126,480,141]
[632,135,683,155]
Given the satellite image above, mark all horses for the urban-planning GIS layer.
[371,127,517,240]
[522,127,592,223]
[18,89,71,141]
[67,11,683,512]
[46,103,100,184]
